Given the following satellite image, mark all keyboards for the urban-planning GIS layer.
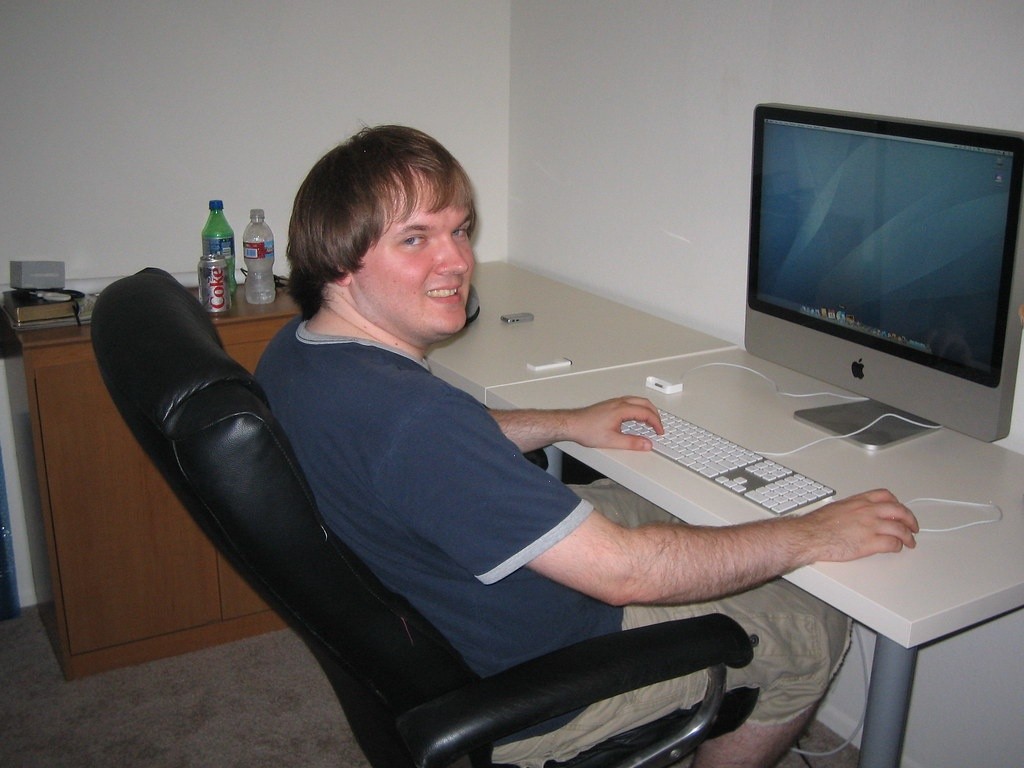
[617,407,837,516]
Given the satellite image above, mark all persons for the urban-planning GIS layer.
[251,123,919,768]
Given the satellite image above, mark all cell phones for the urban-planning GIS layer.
[501,312,533,323]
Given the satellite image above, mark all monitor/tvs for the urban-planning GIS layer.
[744,102,1024,450]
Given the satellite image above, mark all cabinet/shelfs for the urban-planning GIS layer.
[0,284,302,683]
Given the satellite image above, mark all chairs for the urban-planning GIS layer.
[88,267,760,768]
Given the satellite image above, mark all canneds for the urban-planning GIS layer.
[198,254,231,312]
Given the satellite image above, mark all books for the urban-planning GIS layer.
[2,290,77,331]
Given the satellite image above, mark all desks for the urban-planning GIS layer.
[425,260,738,404]
[485,348,1024,768]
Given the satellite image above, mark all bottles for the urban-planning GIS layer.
[243,209,277,305]
[201,199,237,297]
